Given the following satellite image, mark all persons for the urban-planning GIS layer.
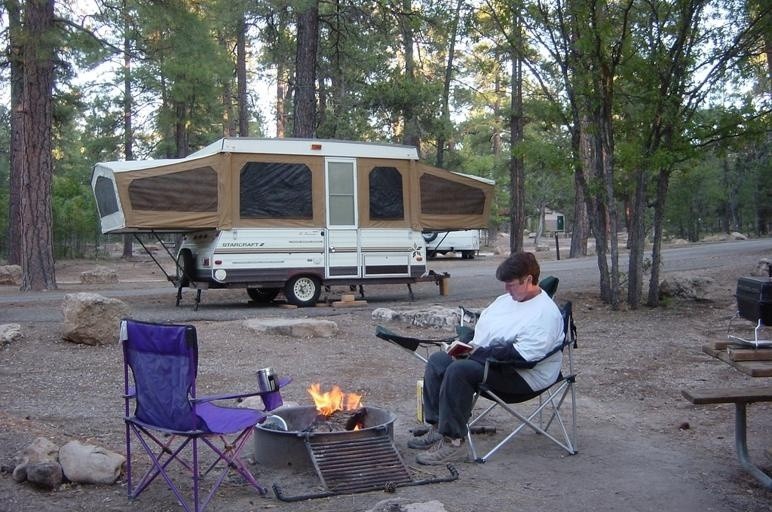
[407,252,564,466]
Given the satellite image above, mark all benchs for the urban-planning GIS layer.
[681,385,772,490]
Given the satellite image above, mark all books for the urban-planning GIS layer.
[442,339,473,358]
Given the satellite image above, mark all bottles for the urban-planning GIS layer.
[255,366,280,395]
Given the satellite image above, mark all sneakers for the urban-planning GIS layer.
[416,438,468,466]
[408,424,444,449]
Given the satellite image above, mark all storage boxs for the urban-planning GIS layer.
[738,275,771,326]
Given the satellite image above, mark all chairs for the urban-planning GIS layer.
[120,318,292,512]
[448,299,577,463]
[376,276,558,363]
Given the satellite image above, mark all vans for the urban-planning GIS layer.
[422,229,481,260]
[90,137,500,306]
